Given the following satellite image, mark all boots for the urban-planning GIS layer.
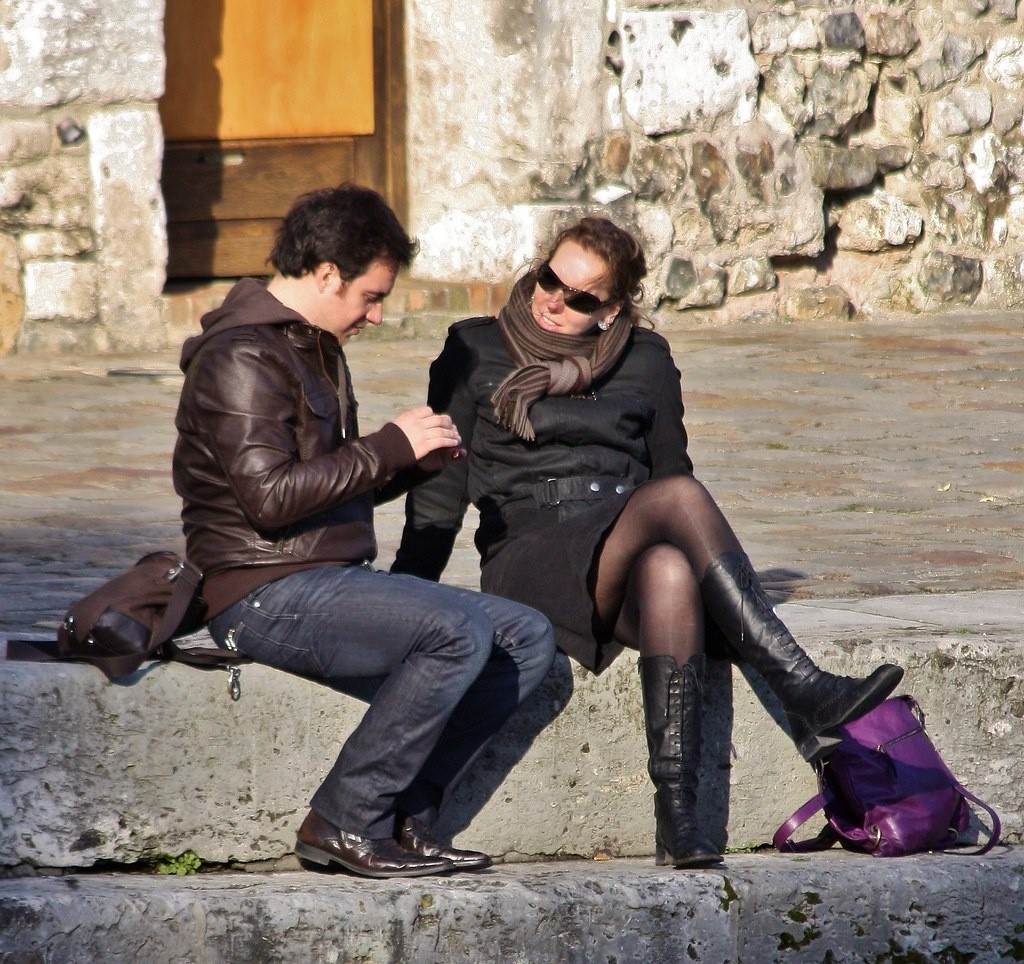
[702,552,905,763]
[637,651,727,866]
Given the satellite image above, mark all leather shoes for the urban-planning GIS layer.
[388,809,494,869]
[295,806,455,878]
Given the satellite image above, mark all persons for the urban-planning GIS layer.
[389,219,905,866]
[173,184,556,876]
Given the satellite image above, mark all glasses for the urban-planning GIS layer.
[536,262,621,316]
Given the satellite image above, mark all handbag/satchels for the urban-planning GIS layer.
[10,551,238,678]
[773,693,1001,855]
